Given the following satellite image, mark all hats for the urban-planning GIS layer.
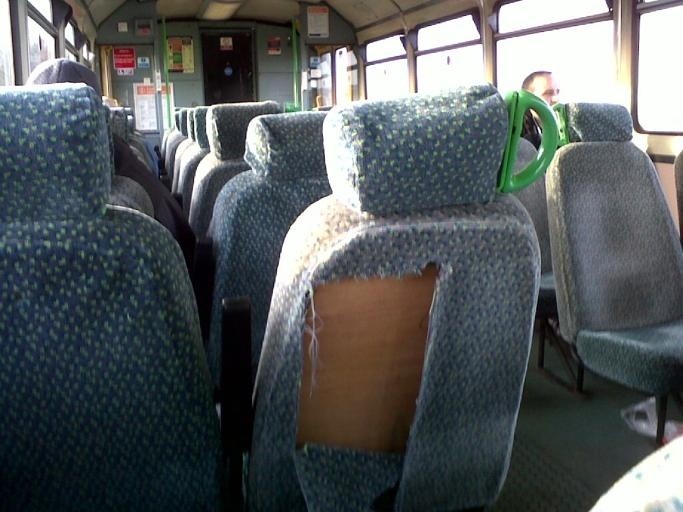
[25,58,102,102]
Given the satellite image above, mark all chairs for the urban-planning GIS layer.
[544,101,683,445]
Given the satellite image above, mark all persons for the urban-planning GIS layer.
[26,58,203,279]
[520,71,560,150]
